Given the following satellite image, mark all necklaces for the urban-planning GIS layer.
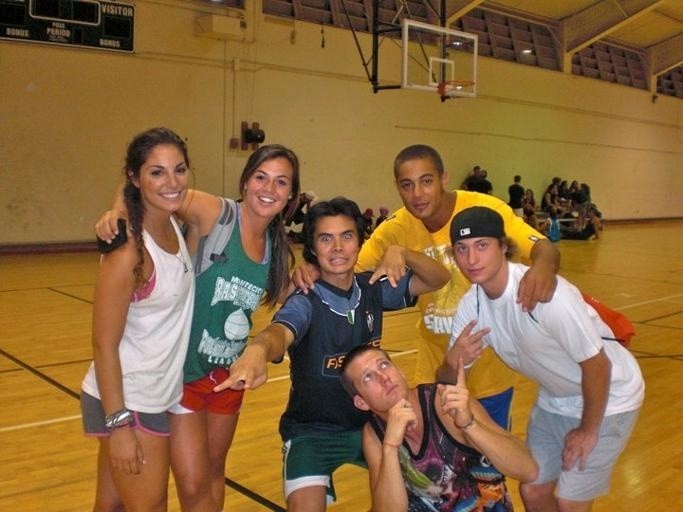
[141,216,188,273]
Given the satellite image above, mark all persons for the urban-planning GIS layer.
[211,196,451,511]
[463,164,603,242]
[94,141,300,510]
[80,127,196,510]
[342,344,539,512]
[362,208,374,238]
[281,189,315,243]
[376,207,388,225]
[292,144,559,432]
[435,208,645,512]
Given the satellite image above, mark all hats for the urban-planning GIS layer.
[452,207,510,258]
[366,209,374,218]
[306,191,319,202]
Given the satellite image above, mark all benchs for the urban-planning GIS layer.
[534,210,605,232]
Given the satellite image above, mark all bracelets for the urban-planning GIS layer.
[104,409,134,431]
[384,442,401,449]
[454,413,475,429]
